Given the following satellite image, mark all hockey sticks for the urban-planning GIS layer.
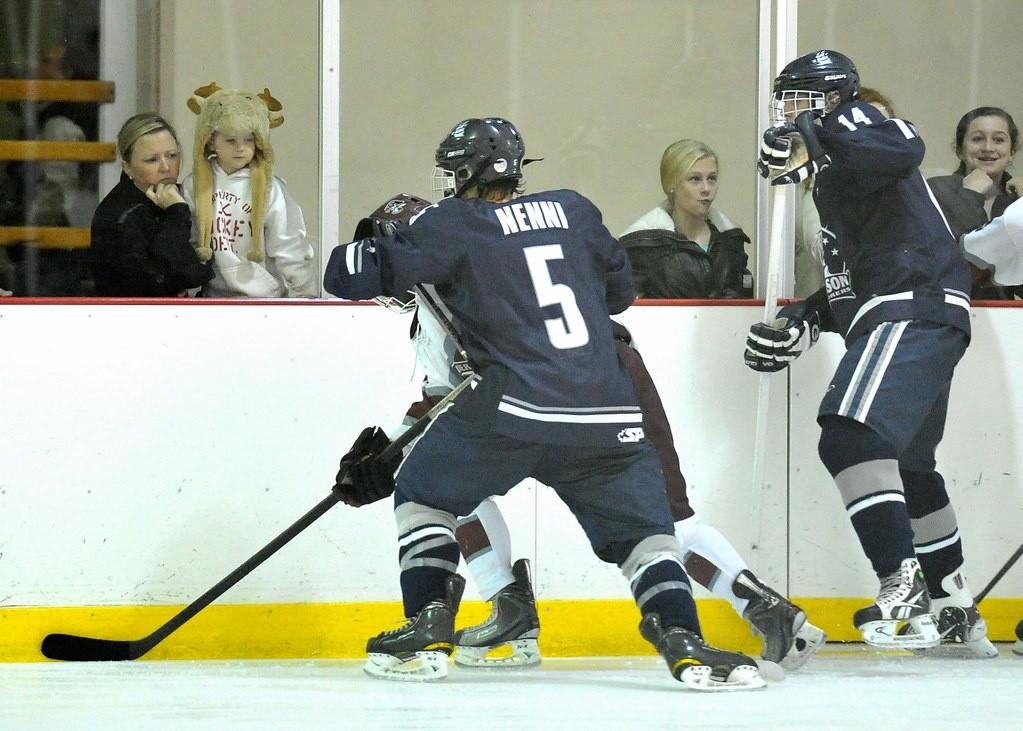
[897,543,1023,656]
[749,135,792,636]
[411,282,468,361]
[41,372,476,662]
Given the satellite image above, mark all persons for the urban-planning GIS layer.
[616,138,756,299]
[181,82,323,298]
[321,116,827,694]
[91,112,216,298]
[22,34,101,301]
[745,51,999,657]
[800,87,1023,302]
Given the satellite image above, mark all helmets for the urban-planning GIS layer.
[353,193,432,237]
[770,50,861,126]
[435,117,525,193]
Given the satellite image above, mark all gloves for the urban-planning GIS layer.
[756,108,832,187]
[743,300,823,373]
[331,426,405,508]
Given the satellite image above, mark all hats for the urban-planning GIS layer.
[186,81,285,264]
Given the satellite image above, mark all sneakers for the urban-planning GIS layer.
[363,574,466,680]
[639,612,767,692]
[452,557,543,669]
[928,560,999,659]
[731,569,826,674]
[853,557,943,650]
